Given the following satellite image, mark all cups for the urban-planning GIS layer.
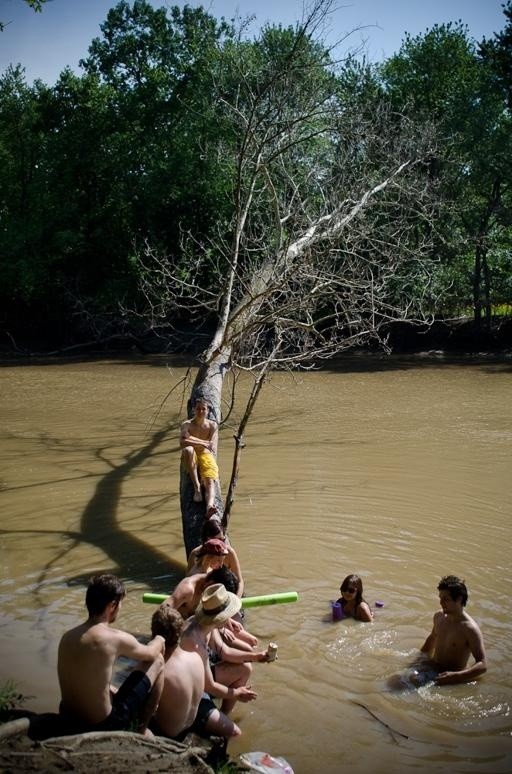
[342,586,359,594]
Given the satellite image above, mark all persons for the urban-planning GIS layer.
[387,573,489,690]
[175,396,221,521]
[133,603,206,740]
[164,519,279,741]
[333,572,376,624]
[56,572,168,737]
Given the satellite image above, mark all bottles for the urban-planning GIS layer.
[196,583,242,626]
[201,540,228,556]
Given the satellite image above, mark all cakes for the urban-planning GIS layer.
[267,643,278,663]
